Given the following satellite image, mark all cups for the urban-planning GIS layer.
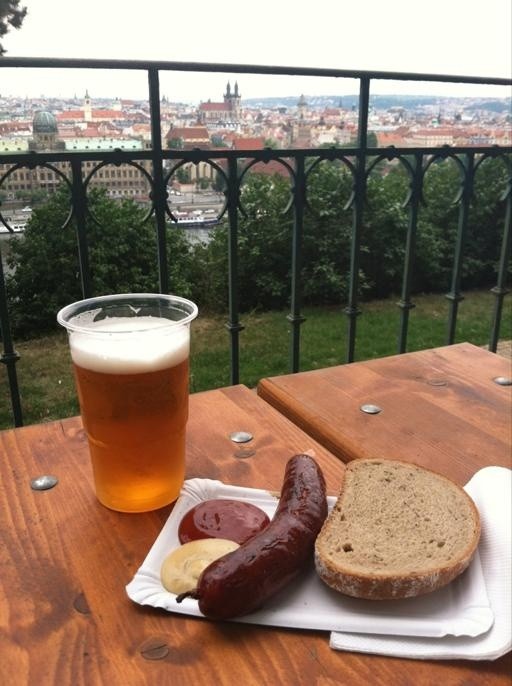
[56,293,198,514]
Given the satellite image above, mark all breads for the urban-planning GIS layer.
[313,458,481,600]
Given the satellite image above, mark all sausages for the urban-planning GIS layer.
[177,455,328,620]
